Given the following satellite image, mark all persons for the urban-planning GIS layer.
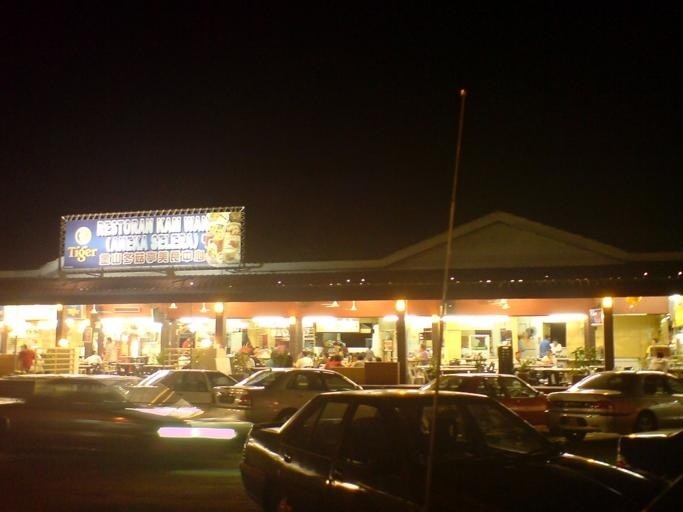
[643,338,658,358]
[16,343,35,372]
[647,352,668,374]
[49,324,565,375]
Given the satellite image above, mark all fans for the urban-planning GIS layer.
[345,301,360,312]
[198,303,211,313]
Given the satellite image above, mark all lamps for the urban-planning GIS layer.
[168,303,178,309]
[88,304,98,314]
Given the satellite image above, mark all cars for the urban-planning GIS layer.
[239,388,682,512]
[416,372,565,438]
[0,373,255,479]
[212,367,378,429]
[544,370,682,444]
[616,425,682,483]
[142,368,243,422]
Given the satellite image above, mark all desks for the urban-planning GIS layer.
[80,355,683,384]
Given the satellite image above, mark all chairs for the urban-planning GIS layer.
[80,355,683,384]
